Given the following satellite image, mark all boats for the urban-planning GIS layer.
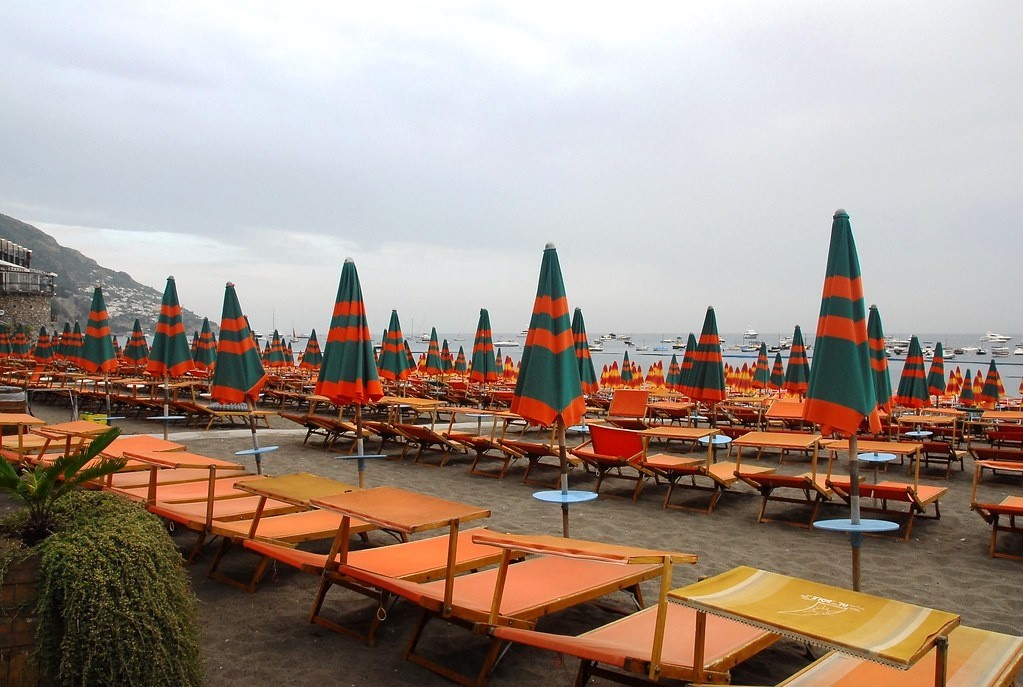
[741,337,811,352]
[718,337,725,342]
[594,333,630,344]
[884,336,956,359]
[454,338,464,341]
[297,333,309,338]
[290,338,298,342]
[624,341,667,351]
[588,344,603,351]
[976,346,1009,357]
[517,331,529,338]
[493,342,519,347]
[953,350,963,354]
[254,332,263,337]
[672,344,687,350]
[421,335,431,341]
[1013,345,1023,355]
[744,330,759,338]
[269,332,283,338]
[979,334,1012,343]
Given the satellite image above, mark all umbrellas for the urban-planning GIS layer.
[0,208,1023,592]
[801,209,885,592]
[315,256,384,489]
[211,281,270,475]
[75,283,119,425]
[143,274,195,442]
[510,243,586,537]
[684,307,727,465]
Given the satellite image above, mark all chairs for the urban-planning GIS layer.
[0,359,1023,687]
